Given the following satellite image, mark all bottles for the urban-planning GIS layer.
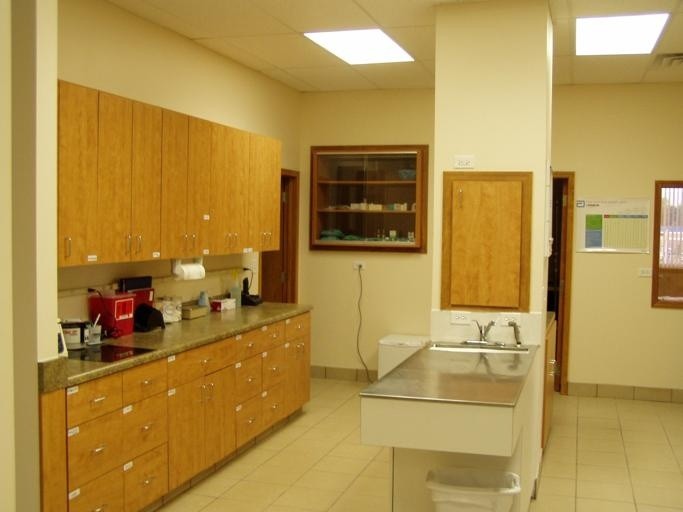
[197,290,210,313]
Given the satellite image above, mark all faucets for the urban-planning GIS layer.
[484,320,498,341]
[508,321,523,344]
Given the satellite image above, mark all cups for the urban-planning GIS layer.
[85,324,101,345]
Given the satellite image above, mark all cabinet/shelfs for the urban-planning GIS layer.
[167,335,235,492]
[443,171,532,313]
[285,309,311,417]
[98,94,161,264]
[217,121,249,256]
[162,108,217,259]
[57,78,98,268]
[39,355,167,511]
[234,319,285,448]
[543,311,558,445]
[249,132,281,252]
[310,143,429,256]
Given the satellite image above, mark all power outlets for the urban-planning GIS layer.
[353,260,366,271]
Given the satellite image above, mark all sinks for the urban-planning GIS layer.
[430,342,530,353]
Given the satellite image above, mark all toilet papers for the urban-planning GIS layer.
[175,263,207,281]
